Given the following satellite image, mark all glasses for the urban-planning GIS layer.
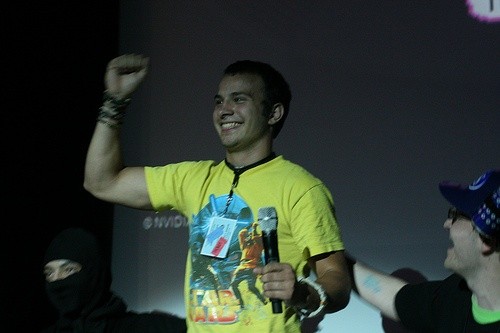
[447,206,472,225]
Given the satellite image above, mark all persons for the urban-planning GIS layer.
[83,54,351,333]
[347,168,500,333]
[40,229,187,333]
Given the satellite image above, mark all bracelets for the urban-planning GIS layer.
[95,92,132,128]
[292,277,328,322]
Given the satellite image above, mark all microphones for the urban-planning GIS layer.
[257,207,282,314]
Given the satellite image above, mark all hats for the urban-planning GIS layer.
[41,226,100,264]
[438,169,500,240]
